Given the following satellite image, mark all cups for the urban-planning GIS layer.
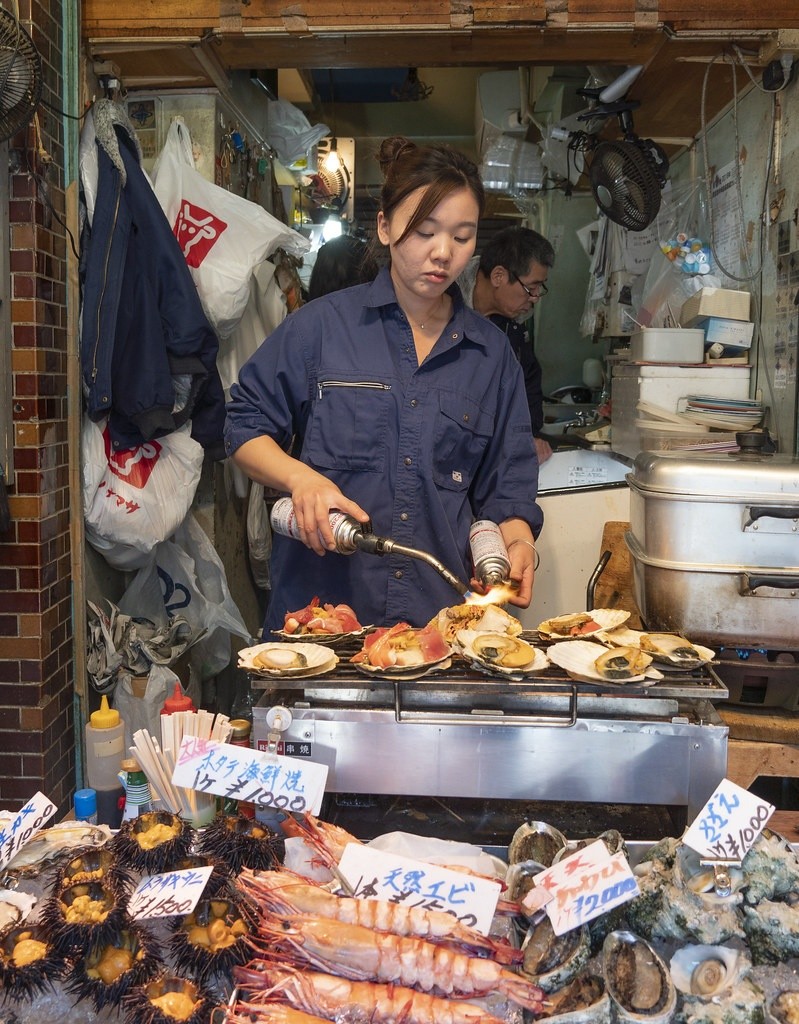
[152,799,162,812]
[163,800,217,830]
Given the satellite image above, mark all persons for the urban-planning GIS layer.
[307,235,379,302]
[457,227,555,463]
[222,144,544,637]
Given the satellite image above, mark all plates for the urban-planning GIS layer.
[679,394,764,431]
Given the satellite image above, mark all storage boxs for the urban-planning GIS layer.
[696,317,755,348]
[630,328,705,363]
[611,366,751,455]
[681,287,750,324]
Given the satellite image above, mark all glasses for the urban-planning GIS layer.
[509,272,548,298]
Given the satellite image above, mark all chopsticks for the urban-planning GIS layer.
[128,709,234,813]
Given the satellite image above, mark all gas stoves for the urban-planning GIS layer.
[639,616,799,707]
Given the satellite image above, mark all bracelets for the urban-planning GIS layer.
[506,540,540,571]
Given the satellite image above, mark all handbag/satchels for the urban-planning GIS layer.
[107,511,257,760]
[151,117,312,343]
[82,412,206,573]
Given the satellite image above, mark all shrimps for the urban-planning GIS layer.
[225,874,548,1015]
[229,957,508,1024]
[209,987,337,1024]
[281,809,519,914]
[228,853,525,966]
[304,855,509,893]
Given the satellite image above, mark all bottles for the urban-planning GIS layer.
[118,758,154,831]
[160,684,197,716]
[271,497,468,596]
[85,696,126,827]
[469,520,511,586]
[221,719,255,820]
[75,789,98,826]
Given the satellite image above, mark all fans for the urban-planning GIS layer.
[0,7,43,144]
[304,150,351,210]
[576,85,670,232]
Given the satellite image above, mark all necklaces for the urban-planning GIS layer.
[421,306,440,328]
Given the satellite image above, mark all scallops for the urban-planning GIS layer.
[237,604,719,691]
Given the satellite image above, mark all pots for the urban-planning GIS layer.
[625,432,799,652]
